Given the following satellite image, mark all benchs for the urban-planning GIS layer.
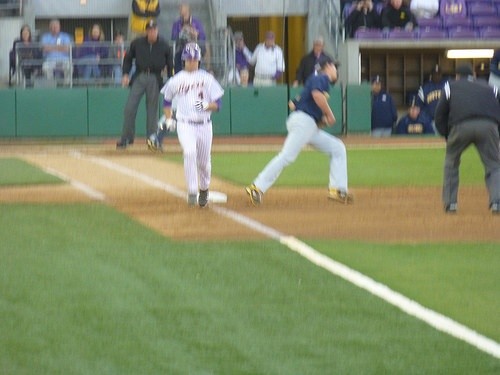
[9,40,226,88]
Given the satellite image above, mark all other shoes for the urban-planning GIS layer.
[491,202,500,212]
[116,138,134,149]
[447,203,456,213]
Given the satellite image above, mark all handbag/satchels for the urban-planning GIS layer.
[156,73,164,90]
[129,69,141,86]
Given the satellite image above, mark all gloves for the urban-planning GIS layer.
[275,71,281,79]
[194,100,209,111]
[165,118,176,132]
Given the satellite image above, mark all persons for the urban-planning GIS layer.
[244,54,356,206]
[229,30,285,87]
[292,36,338,92]
[131,0,160,37]
[432,66,500,214]
[159,42,227,209]
[116,19,173,150]
[342,0,419,37]
[369,62,499,138]
[169,4,206,73]
[11,16,137,89]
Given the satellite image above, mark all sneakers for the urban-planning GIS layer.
[187,195,198,207]
[198,189,208,208]
[327,187,353,203]
[245,183,262,207]
[147,137,164,153]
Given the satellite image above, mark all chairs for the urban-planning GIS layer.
[342,0,500,41]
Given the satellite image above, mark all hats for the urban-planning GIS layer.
[408,95,420,107]
[266,33,275,40]
[456,65,473,76]
[315,55,334,70]
[432,65,442,72]
[146,21,156,29]
[373,75,382,82]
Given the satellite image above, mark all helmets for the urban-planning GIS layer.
[180,43,200,60]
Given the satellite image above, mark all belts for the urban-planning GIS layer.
[142,69,153,73]
[189,119,210,125]
